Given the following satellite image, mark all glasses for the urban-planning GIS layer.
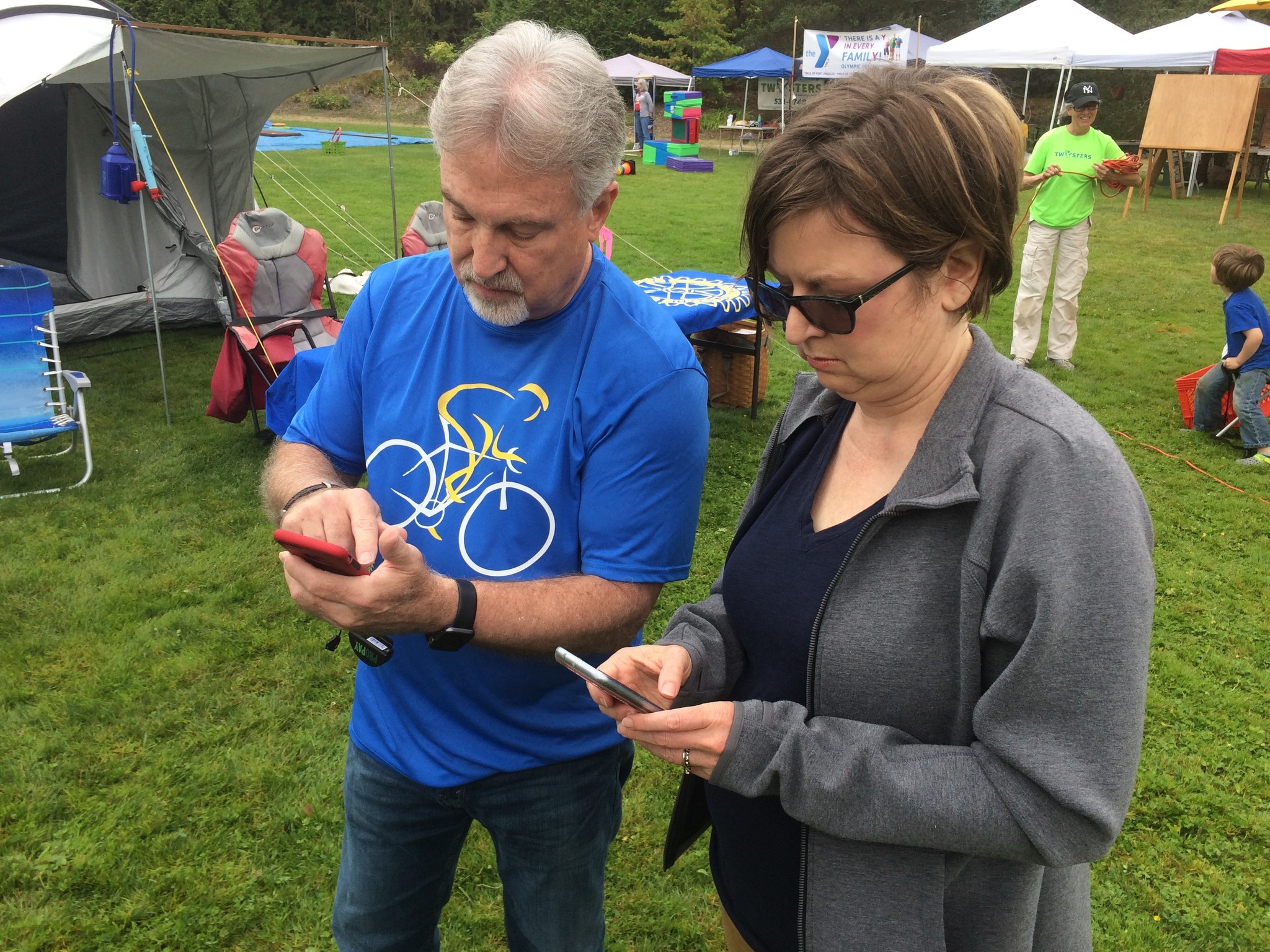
[742,260,923,337]
[1070,101,1100,112]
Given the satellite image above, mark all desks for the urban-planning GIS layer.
[1183,146,1270,197]
[718,126,777,156]
[632,269,781,420]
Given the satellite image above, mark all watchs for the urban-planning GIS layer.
[425,580,477,653]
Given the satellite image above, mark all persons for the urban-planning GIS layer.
[634,79,653,150]
[1010,82,1142,371]
[884,38,890,60]
[895,36,903,61]
[259,19,710,952]
[889,34,897,61]
[1180,244,1270,469]
[554,64,1156,952]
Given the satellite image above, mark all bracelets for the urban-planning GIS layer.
[277,481,349,527]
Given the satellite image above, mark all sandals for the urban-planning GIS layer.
[1235,453,1270,469]
[1179,427,1222,439]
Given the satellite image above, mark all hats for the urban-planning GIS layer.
[1068,81,1101,107]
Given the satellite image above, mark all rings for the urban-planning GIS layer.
[683,749,691,774]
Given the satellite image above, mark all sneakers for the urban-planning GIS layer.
[1045,356,1075,372]
[1011,355,1030,368]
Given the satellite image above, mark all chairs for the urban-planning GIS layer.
[737,122,760,155]
[400,200,448,258]
[0,265,93,498]
[205,207,347,442]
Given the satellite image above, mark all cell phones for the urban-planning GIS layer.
[553,646,665,714]
[272,529,370,576]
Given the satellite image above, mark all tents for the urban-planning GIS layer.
[41,0,398,346]
[687,47,793,155]
[1049,10,1270,197]
[865,24,946,62]
[925,0,1169,131]
[1186,47,1270,197]
[790,55,834,80]
[601,53,695,140]
[1209,0,1270,13]
[0,0,172,428]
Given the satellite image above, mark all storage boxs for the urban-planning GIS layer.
[693,320,773,409]
[321,141,347,155]
[1175,364,1270,431]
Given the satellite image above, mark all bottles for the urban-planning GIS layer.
[757,114,762,127]
[727,113,733,126]
